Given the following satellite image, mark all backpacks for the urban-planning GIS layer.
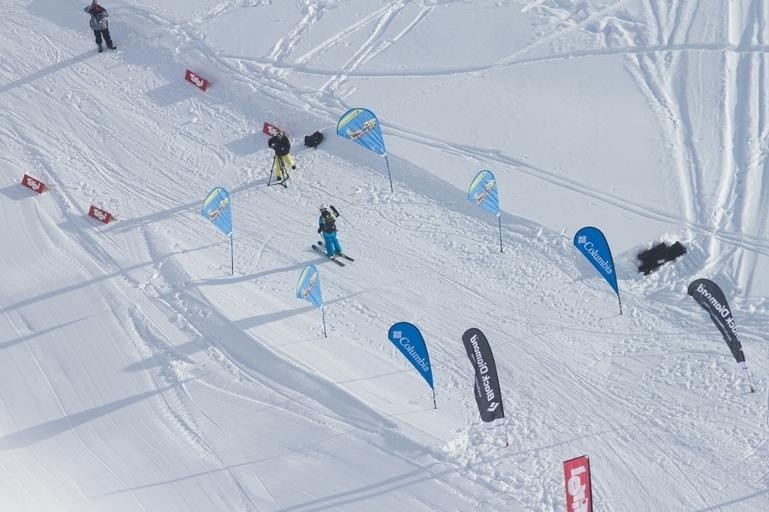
[322,213,337,233]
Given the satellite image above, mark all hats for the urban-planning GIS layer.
[94,4,102,12]
[317,203,328,210]
[275,129,284,136]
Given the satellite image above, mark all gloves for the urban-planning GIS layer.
[329,205,334,209]
[318,228,322,233]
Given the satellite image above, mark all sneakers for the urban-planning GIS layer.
[109,46,117,50]
[291,165,297,170]
[276,176,282,181]
[97,45,103,53]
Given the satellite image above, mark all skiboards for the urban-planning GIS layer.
[312,241,354,267]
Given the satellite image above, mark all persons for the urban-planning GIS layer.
[268,128,296,180]
[83,0,116,52]
[317,203,343,259]
